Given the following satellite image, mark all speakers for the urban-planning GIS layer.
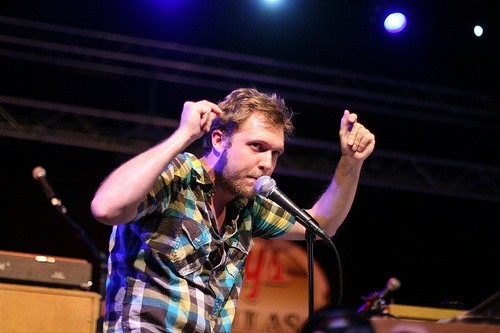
[0,250,102,333]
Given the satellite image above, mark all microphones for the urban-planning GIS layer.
[252,174,375,333]
[355,277,402,319]
[32,164,67,215]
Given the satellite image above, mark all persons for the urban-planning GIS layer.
[91,88,376,333]
